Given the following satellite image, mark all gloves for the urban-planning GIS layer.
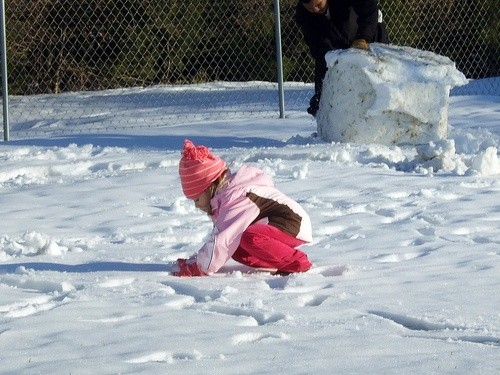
[352,39,369,51]
[173,257,206,277]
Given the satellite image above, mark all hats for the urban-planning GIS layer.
[178,139,226,199]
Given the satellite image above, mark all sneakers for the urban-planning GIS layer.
[271,266,312,279]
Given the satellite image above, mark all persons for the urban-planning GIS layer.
[292,0,387,118]
[169,137,315,277]
